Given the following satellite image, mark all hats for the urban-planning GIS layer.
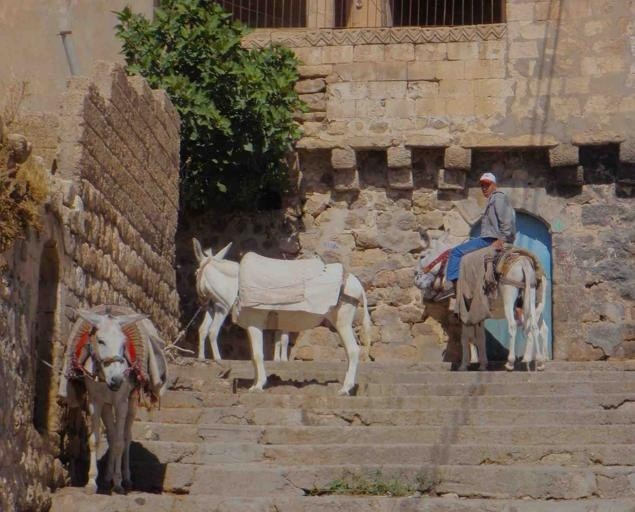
[480,173,496,184]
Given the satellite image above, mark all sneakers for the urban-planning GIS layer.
[434,288,455,302]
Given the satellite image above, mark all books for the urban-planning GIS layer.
[450,197,483,225]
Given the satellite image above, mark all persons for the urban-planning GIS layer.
[429,171,518,304]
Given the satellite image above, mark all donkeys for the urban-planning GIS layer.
[62,307,141,495]
[188,236,373,397]
[413,224,544,372]
